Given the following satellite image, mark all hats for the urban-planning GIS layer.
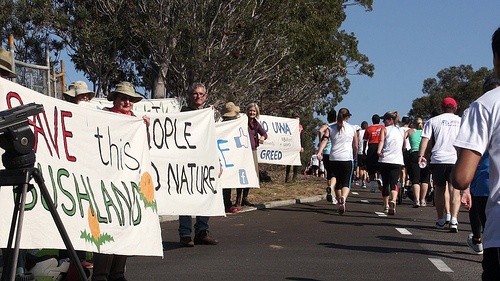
[0,48,17,78]
[222,102,240,117]
[343,112,352,118]
[379,113,396,120]
[63,81,95,102]
[441,97,457,109]
[107,81,143,103]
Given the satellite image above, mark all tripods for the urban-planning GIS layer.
[0,168,89,281]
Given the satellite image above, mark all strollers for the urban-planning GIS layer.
[398,148,433,204]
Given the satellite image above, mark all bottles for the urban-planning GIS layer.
[419,162,427,168]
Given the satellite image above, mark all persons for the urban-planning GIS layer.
[451,27,500,281]
[286,116,304,181]
[418,97,461,232]
[363,114,385,192]
[352,121,376,188]
[377,111,406,215]
[91,81,143,281]
[400,116,435,207]
[305,152,327,178]
[317,108,358,215]
[461,78,500,255]
[178,82,220,247]
[59,81,94,269]
[319,109,339,205]
[222,102,240,213]
[235,103,268,206]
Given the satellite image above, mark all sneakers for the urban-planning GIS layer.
[468,234,483,254]
[446,214,451,222]
[180,237,194,247]
[81,261,93,268]
[383,206,389,213]
[361,181,366,188]
[388,201,395,215]
[420,200,426,207]
[331,197,338,204]
[326,186,333,202]
[413,200,420,208]
[436,219,446,229]
[225,208,240,214]
[338,196,345,214]
[449,222,458,233]
[194,230,218,245]
[377,178,383,191]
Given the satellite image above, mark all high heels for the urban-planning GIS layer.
[241,197,254,206]
[235,197,240,207]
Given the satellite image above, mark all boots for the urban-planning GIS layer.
[291,175,297,183]
[285,173,290,183]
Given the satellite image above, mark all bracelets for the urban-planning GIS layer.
[418,156,425,157]
